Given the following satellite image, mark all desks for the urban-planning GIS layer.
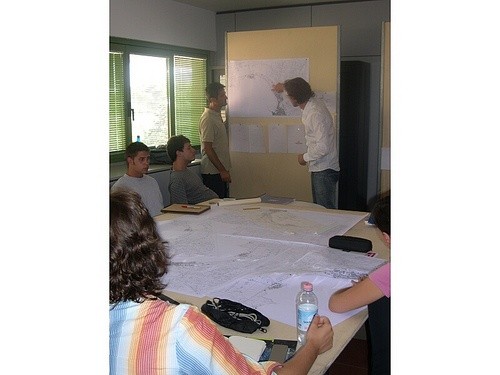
[153,198,391,374]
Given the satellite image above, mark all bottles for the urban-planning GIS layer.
[296,283,319,346]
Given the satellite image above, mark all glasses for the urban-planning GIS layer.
[283,93,292,99]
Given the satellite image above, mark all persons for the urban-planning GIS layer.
[284,77,341,209]
[111,142,165,218]
[199,83,233,198]
[328,194,391,312]
[109,189,334,375]
[167,135,219,205]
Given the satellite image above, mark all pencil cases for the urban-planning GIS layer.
[329,235,372,253]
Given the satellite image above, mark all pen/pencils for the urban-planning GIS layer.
[181,205,201,209]
